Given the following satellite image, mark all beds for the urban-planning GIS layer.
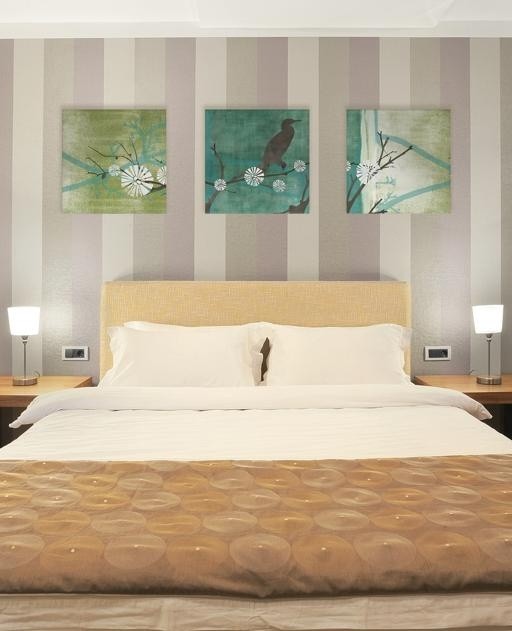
[0,280,511,630]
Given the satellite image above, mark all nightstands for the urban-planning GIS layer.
[414,374,512,439]
[0,370,93,437]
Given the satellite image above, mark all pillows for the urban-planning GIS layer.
[100,320,263,386]
[264,322,414,389]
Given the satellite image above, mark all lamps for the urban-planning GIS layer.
[7,307,37,388]
[471,301,504,387]
